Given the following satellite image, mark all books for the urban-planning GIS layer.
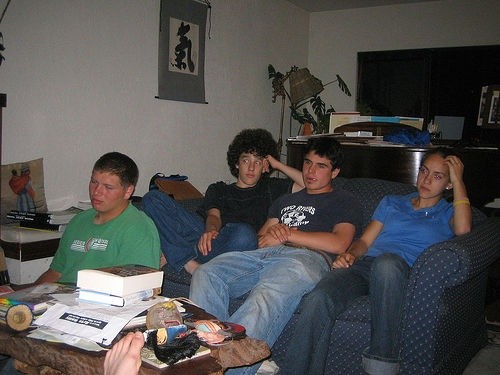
[0,264,186,330]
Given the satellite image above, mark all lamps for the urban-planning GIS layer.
[273,69,324,180]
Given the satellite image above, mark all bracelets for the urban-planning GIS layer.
[453,197,469,201]
[453,201,470,206]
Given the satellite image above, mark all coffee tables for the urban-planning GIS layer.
[0,280,272,375]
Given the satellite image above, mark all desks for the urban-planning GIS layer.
[284,136,500,213]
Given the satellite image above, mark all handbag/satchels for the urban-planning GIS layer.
[150,173,204,199]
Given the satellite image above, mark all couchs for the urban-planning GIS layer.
[138,175,500,375]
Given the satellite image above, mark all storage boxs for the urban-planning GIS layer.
[0,222,65,285]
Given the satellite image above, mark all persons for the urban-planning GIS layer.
[141,129,305,277]
[104,331,144,375]
[275,147,473,375]
[32,152,161,285]
[189,137,359,375]
